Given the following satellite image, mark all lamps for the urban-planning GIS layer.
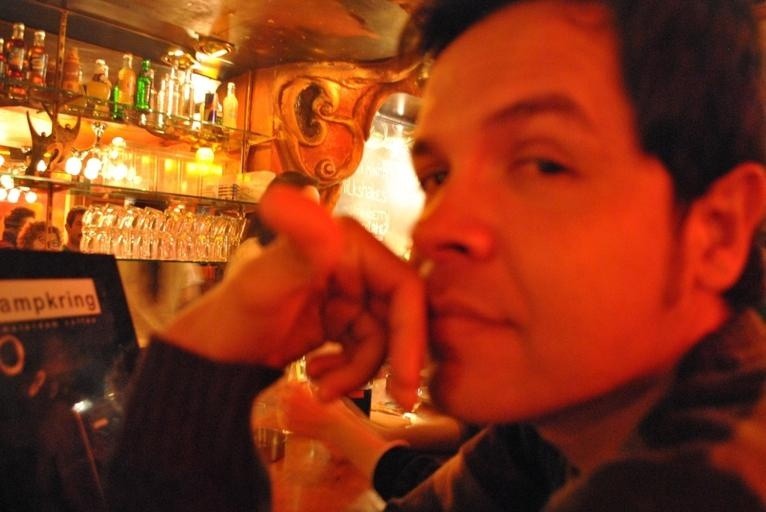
[0,99,145,210]
[163,37,239,78]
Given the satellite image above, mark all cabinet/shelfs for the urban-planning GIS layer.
[0,0,266,267]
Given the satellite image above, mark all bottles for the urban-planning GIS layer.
[275,355,316,465]
[0,21,239,136]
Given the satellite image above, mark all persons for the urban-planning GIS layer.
[222,168,322,383]
[102,0,766,511]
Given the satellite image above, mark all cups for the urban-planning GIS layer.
[80,199,246,258]
